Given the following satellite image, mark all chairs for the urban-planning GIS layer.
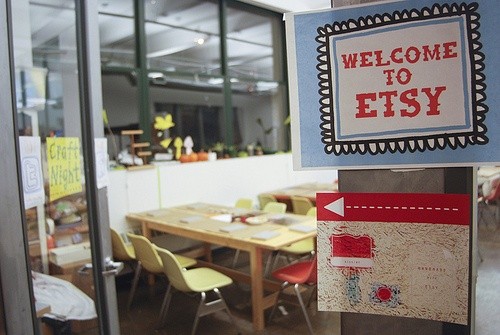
[478,178,500,224]
[110,227,196,309]
[267,254,317,335]
[151,244,237,335]
[235,194,316,216]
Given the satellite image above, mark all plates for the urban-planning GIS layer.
[246,216,267,224]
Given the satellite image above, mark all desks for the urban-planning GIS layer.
[125,202,317,332]
[269,185,338,199]
